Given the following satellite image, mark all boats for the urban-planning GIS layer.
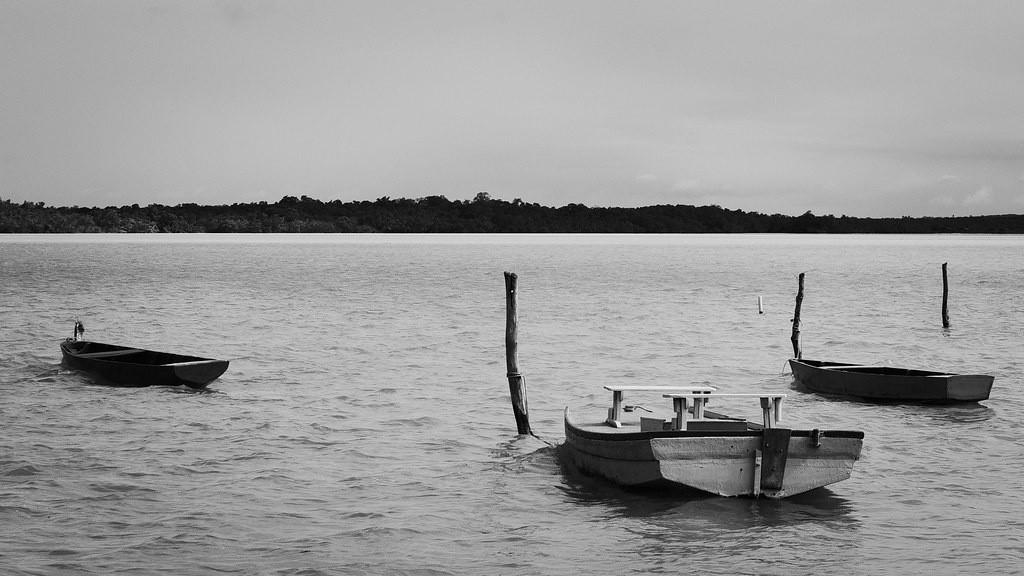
[563,384,866,500]
[788,358,996,404]
[61,338,229,387]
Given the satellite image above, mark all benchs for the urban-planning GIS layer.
[80,349,144,358]
[819,365,882,370]
[663,392,788,431]
[604,385,716,428]
